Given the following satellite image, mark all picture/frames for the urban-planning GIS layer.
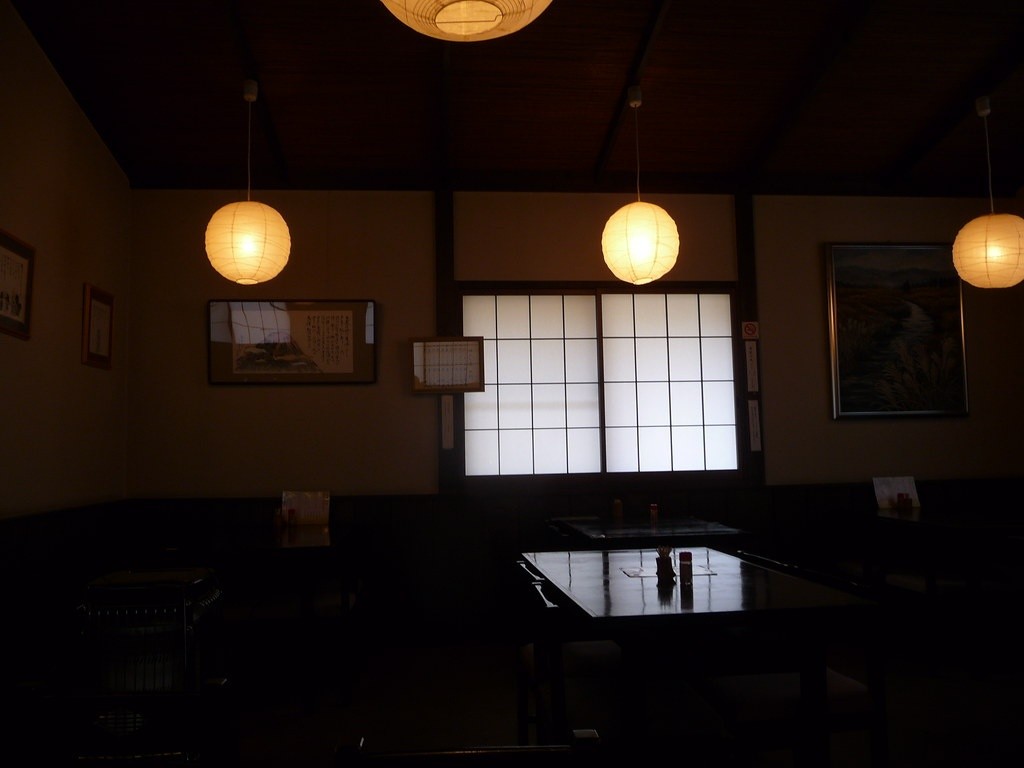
[206,298,379,387]
[0,229,35,342]
[408,335,485,397]
[823,241,969,421]
[82,282,116,371]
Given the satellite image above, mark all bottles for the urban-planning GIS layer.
[681,582,692,609]
[647,503,658,522]
[287,509,297,527]
[897,493,904,509]
[904,494,912,509]
[680,553,692,580]
[612,499,623,518]
[273,512,281,528]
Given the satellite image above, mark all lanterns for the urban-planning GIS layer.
[952,214,1024,288]
[205,201,291,284]
[601,201,679,285]
[385,0,551,42]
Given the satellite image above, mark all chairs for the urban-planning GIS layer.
[59,587,246,768]
[696,559,890,768]
[511,559,622,748]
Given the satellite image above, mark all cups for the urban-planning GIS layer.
[656,557,673,576]
[657,576,672,590]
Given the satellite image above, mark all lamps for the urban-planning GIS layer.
[204,79,292,286]
[600,83,680,286]
[380,0,554,42]
[951,86,1024,289]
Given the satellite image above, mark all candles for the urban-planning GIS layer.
[612,498,624,519]
[646,503,660,521]
[655,544,676,582]
[680,552,694,585]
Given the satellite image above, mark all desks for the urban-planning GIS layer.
[520,548,880,768]
[77,567,225,689]
[543,515,755,548]
